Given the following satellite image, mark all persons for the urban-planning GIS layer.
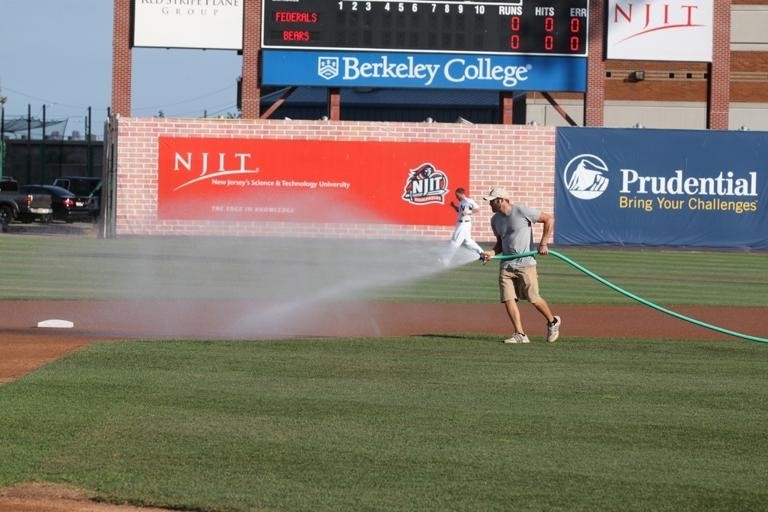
[435,187,487,265]
[479,188,562,344]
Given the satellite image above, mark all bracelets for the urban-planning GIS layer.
[539,242,549,247]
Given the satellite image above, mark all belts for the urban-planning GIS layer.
[458,219,471,223]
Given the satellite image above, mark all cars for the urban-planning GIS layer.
[0,176,101,224]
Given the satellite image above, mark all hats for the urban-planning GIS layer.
[483,186,512,202]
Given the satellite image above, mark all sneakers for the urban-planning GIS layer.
[502,333,531,346]
[437,257,488,267]
[547,313,562,343]
